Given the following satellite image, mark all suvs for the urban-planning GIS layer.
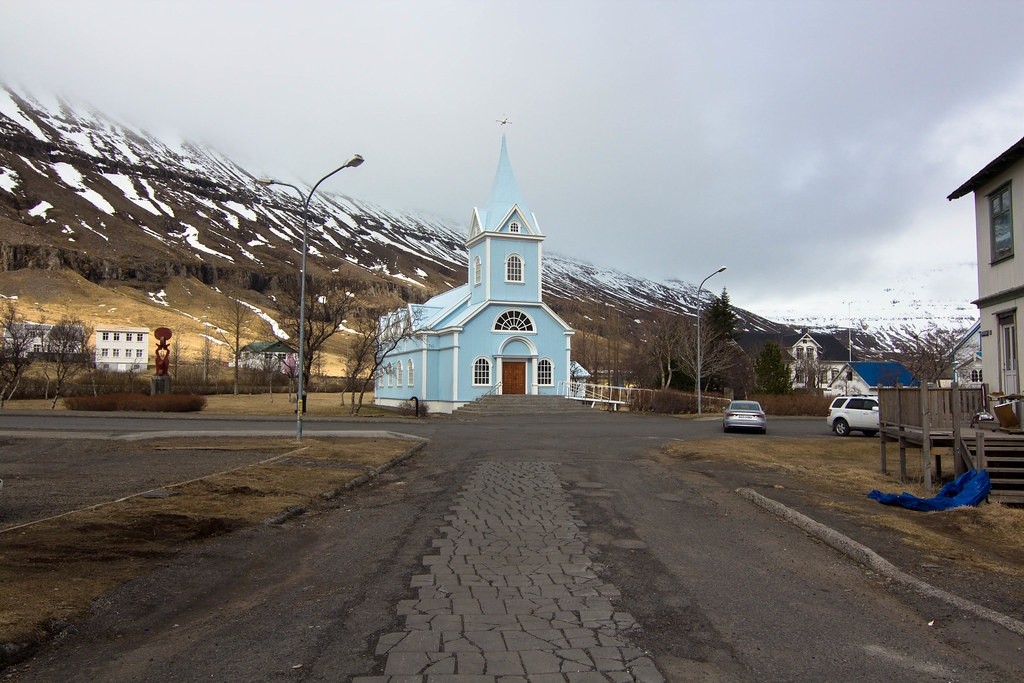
[827,395,882,436]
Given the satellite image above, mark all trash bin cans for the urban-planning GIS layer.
[296,390,307,415]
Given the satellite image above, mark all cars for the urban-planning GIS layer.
[723,400,767,433]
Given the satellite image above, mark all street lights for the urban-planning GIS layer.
[255,154,365,442]
[697,266,727,415]
